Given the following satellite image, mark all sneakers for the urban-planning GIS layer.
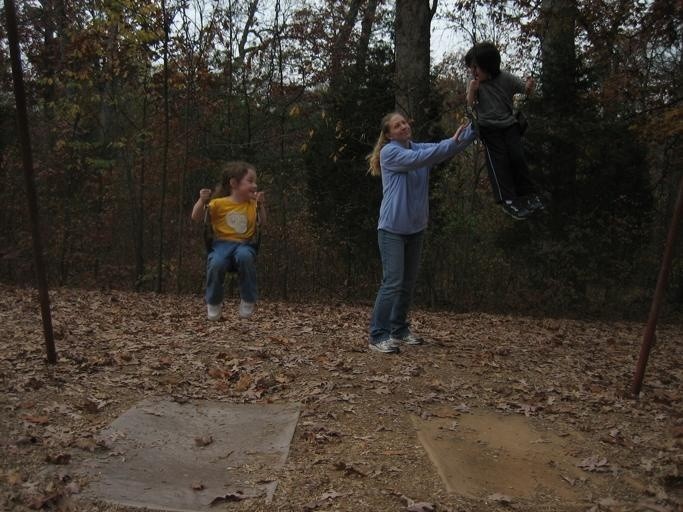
[369,326,423,354]
[206,302,223,322]
[239,296,255,318]
[502,195,546,221]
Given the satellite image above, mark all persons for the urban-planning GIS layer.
[368,112,477,354]
[465,42,544,221]
[191,162,267,321]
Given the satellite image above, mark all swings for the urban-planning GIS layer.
[471,0,558,221]
[180,0,280,270]
